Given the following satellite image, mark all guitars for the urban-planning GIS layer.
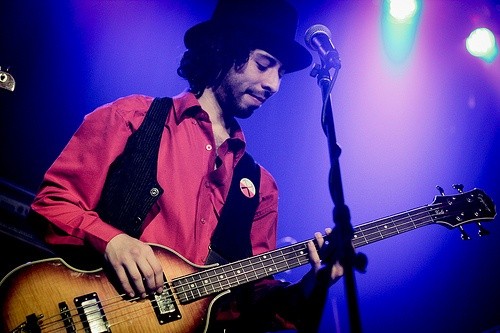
[1,185,497,333]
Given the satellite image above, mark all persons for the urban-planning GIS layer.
[29,2,345,333]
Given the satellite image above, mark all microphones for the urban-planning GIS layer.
[305,24,342,70]
